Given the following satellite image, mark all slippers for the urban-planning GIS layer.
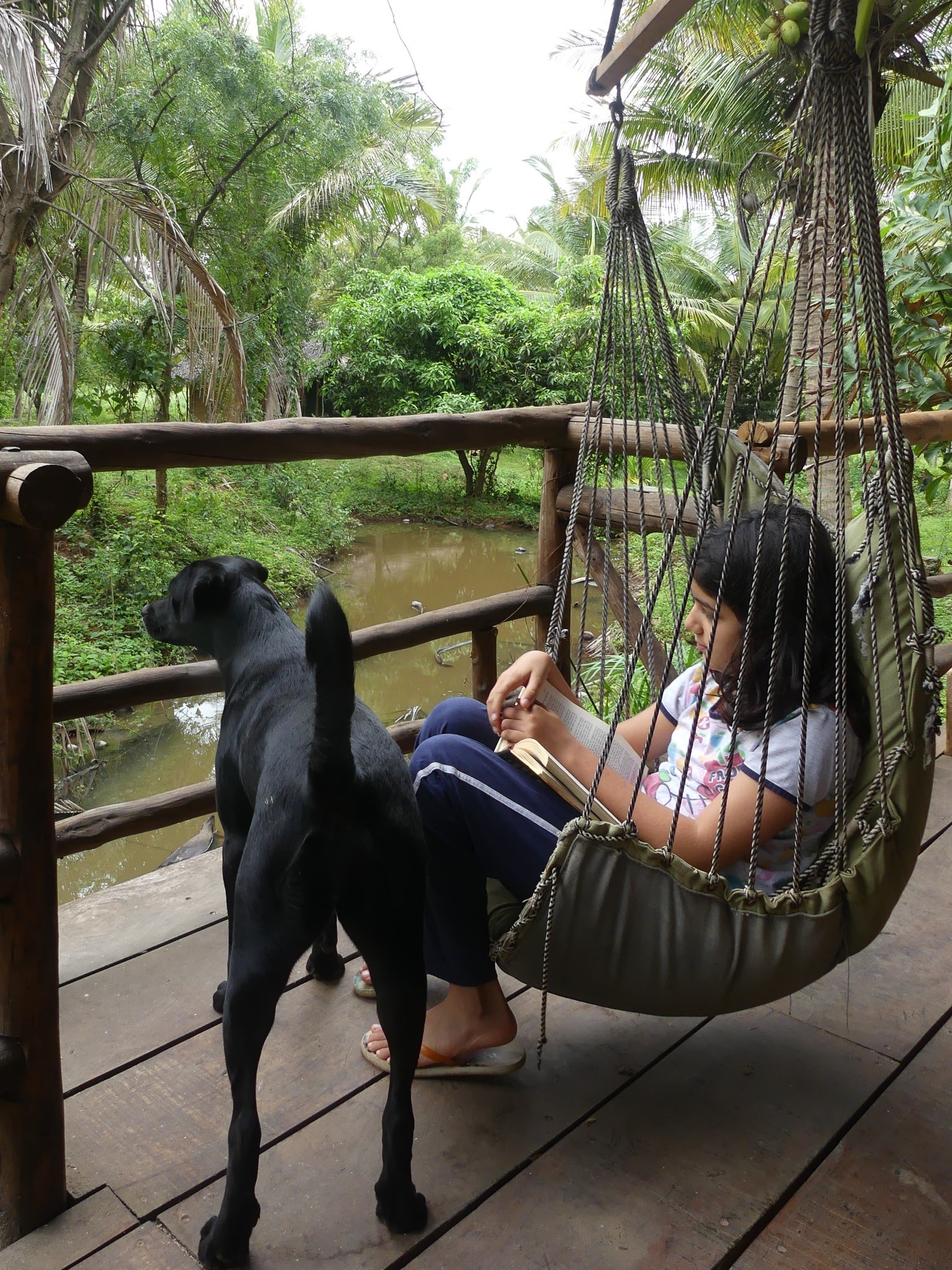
[353,966,377,998]
[360,1024,527,1077]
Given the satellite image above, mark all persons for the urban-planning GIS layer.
[355,502,873,1076]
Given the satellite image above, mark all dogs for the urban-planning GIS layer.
[146,557,434,1269]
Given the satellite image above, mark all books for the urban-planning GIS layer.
[494,679,649,826]
